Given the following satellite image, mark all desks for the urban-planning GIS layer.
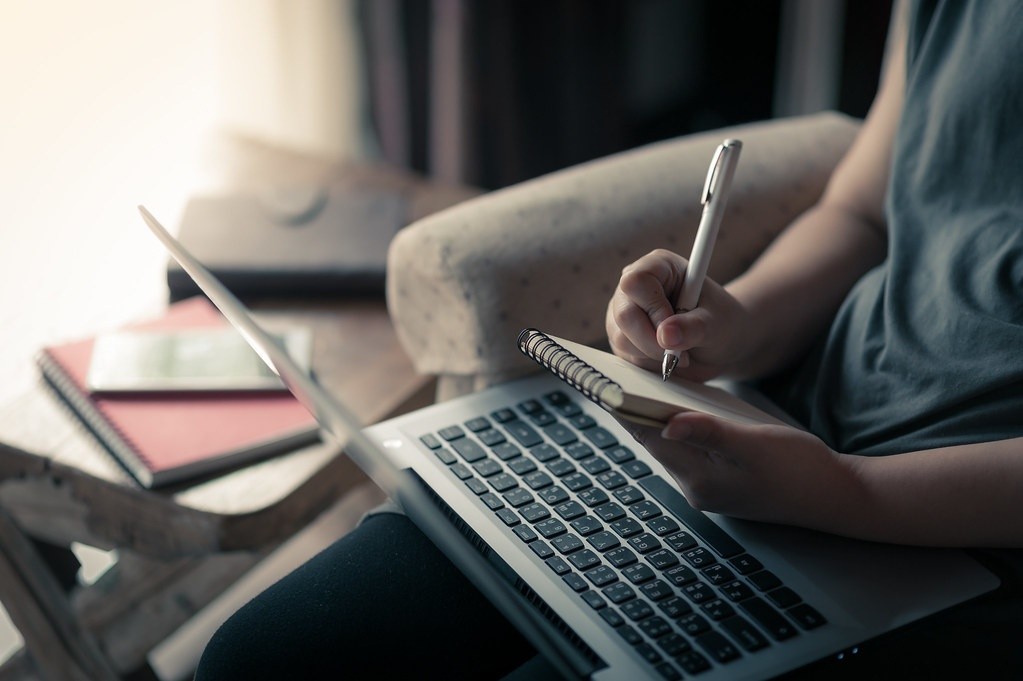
[0,288,440,681]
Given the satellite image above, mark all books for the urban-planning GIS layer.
[516,327,798,430]
[31,294,320,489]
[166,200,409,306]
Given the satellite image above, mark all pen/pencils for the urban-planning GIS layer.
[661,136,745,386]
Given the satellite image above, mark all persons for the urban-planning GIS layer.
[193,0,1023,681]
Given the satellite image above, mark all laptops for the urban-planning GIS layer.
[136,205,997,681]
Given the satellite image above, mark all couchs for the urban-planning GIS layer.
[385,111,858,407]
